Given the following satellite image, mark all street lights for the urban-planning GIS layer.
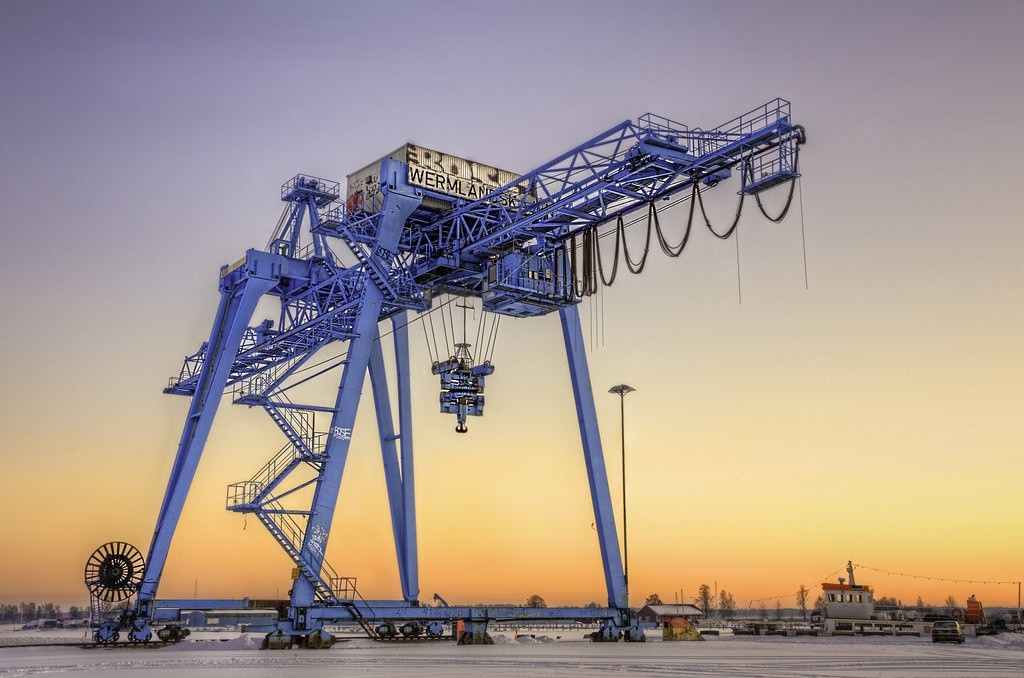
[608,384,637,580]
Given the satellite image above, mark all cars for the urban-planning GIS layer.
[931,620,965,643]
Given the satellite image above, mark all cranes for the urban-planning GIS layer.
[95,94,807,653]
